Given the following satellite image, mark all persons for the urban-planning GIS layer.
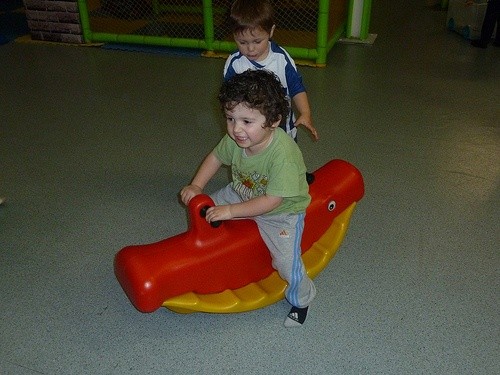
[222,0,319,145]
[178,66,316,329]
